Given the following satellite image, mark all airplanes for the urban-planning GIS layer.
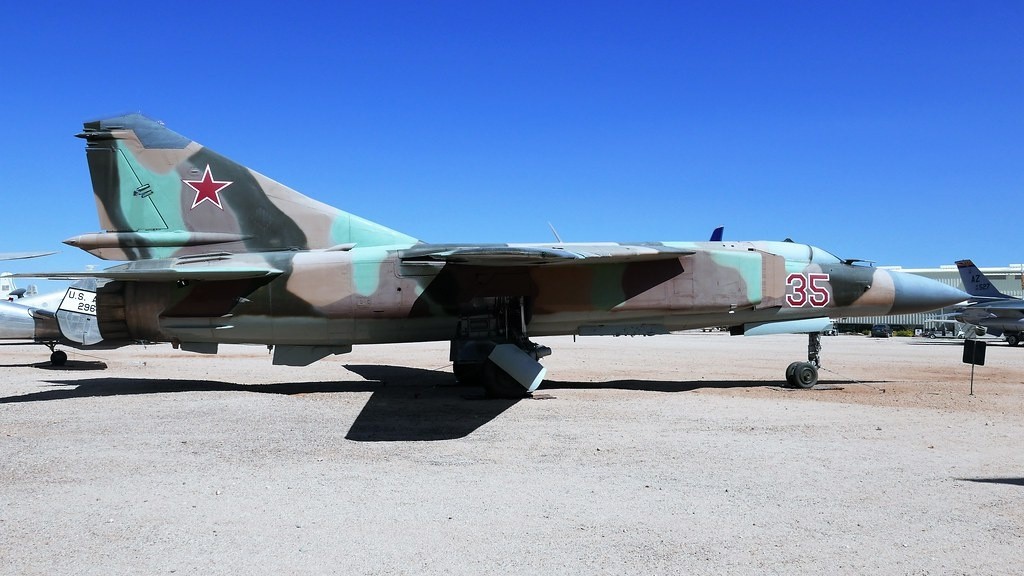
[922,259,1024,347]
[0,112,1007,401]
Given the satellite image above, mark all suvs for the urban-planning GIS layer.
[823,324,839,336]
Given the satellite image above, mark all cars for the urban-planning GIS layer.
[871,324,893,338]
